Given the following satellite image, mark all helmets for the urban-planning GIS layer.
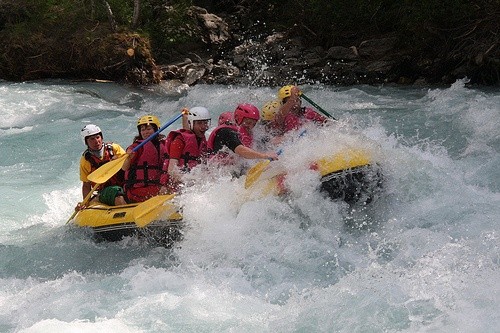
[234,103,259,126]
[278,85,301,102]
[187,106,211,130]
[261,100,282,121]
[219,112,236,127]
[136,115,160,130]
[80,124,103,145]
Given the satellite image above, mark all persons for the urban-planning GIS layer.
[75,86,330,211]
[74,124,128,208]
[122,107,191,203]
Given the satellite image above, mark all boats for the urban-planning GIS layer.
[73,145,383,251]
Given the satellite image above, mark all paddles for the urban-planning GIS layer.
[245,129,306,189]
[66,184,99,226]
[86,110,186,185]
[298,90,337,119]
